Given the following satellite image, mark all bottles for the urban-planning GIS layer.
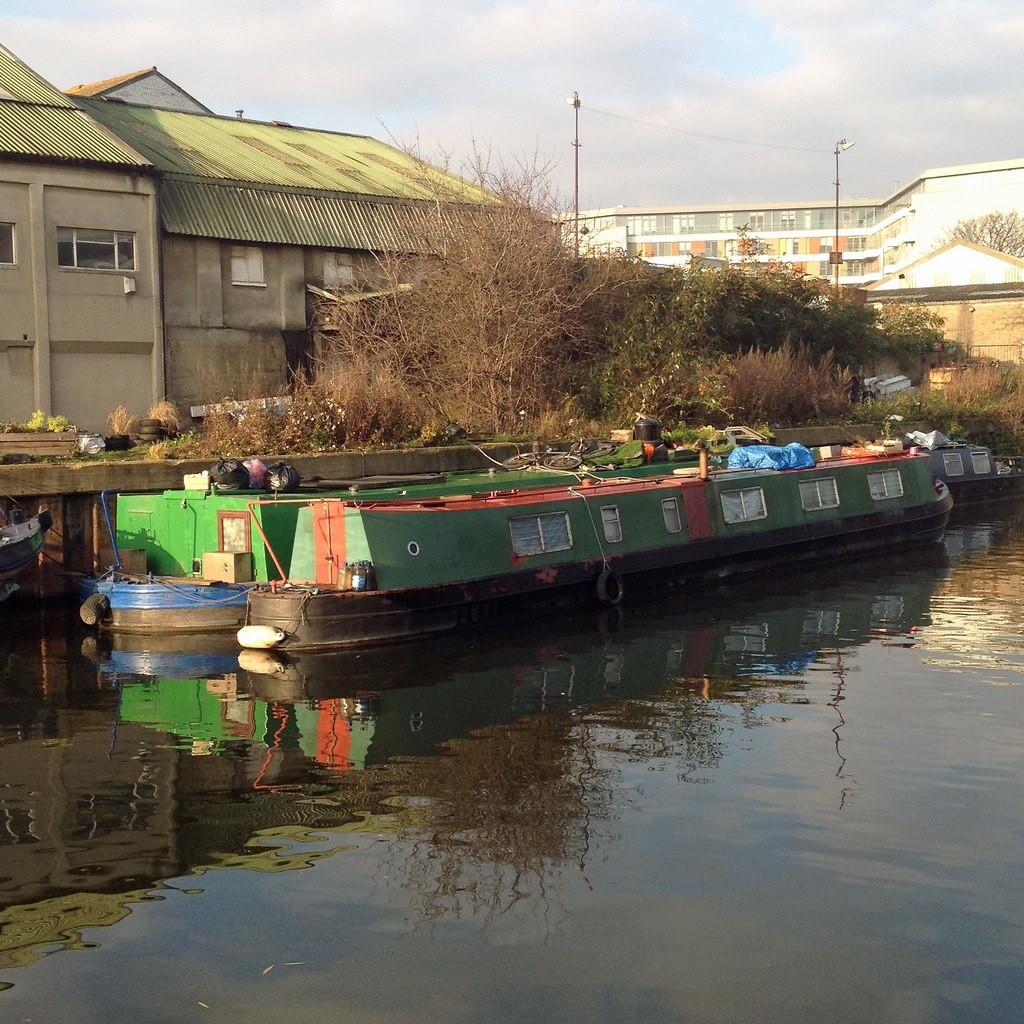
[337,560,374,591]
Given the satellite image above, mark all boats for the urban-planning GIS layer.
[116,499,1024,769]
[73,434,1024,649]
[0,508,53,600]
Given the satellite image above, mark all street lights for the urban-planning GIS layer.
[567,96,590,257]
[837,140,856,286]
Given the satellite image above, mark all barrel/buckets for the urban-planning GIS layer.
[238,649,286,675]
[237,625,285,648]
